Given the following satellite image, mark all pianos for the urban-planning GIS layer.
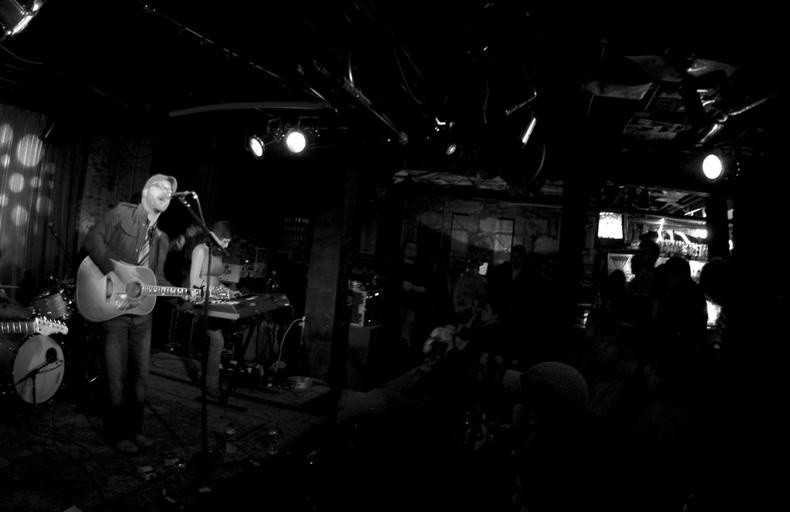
[192,290,291,321]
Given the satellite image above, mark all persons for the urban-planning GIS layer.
[423,231,730,512]
[85,173,178,456]
[190,221,243,403]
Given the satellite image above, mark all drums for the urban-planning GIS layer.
[0,333,66,404]
[31,288,74,320]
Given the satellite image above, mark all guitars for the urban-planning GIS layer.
[76,255,204,322]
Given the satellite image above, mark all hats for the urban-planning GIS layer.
[144,173,178,193]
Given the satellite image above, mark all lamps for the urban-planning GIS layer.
[248,108,288,159]
[0,0,48,36]
[698,136,742,183]
[285,108,329,159]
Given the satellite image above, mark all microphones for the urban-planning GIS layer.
[368,105,408,147]
[166,191,192,198]
